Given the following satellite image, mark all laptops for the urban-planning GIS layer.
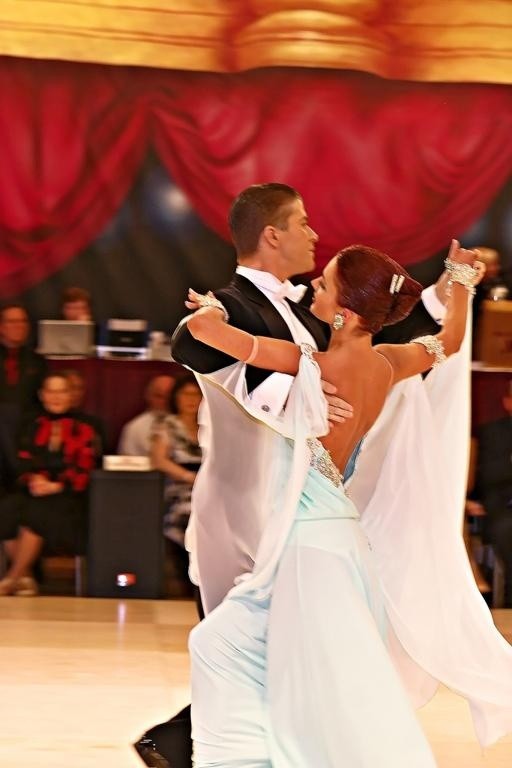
[39,321,94,361]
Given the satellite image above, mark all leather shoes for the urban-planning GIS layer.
[0,576,40,597]
[134,736,173,768]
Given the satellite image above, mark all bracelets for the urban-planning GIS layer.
[196,293,231,323]
[444,256,478,295]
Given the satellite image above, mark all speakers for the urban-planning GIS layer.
[85,469,163,599]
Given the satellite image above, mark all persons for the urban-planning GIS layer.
[473,246,508,331]
[180,248,512,768]
[0,289,106,596]
[117,374,205,593]
[457,380,511,610]
[133,181,481,767]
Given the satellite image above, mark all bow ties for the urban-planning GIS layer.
[273,284,308,303]
